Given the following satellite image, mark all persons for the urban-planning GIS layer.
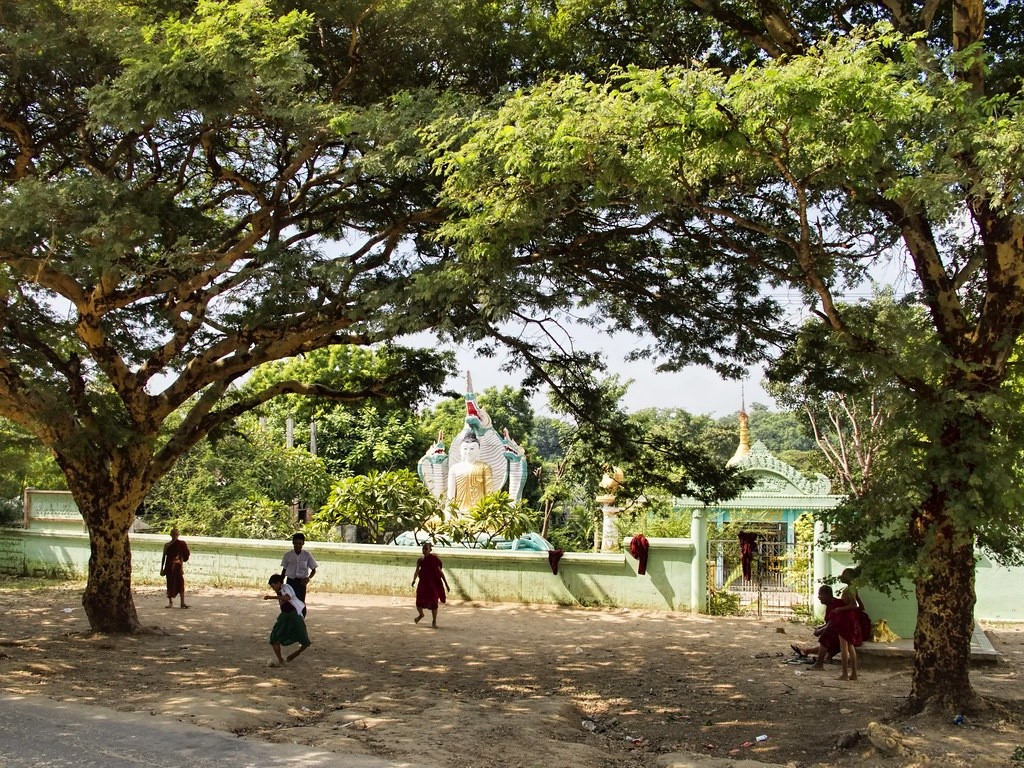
[160,529,191,608]
[264,574,311,666]
[280,533,318,618]
[807,585,845,669]
[445,432,493,524]
[412,543,451,629]
[790,611,870,657]
[833,567,866,682]
